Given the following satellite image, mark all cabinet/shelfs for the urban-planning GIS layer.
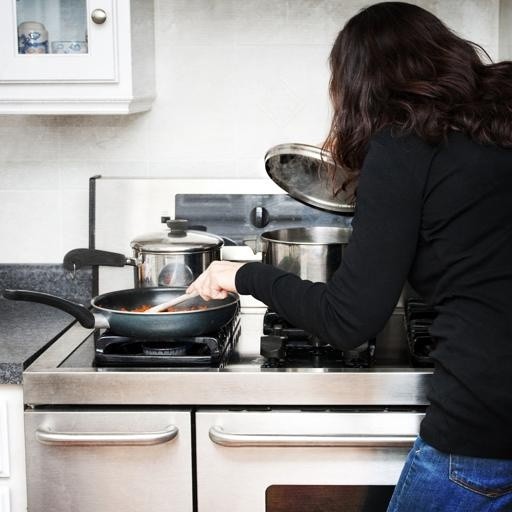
[26,408,197,511]
[2,0,157,116]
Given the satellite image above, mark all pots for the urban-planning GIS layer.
[255,227,354,284]
[63,219,224,289]
[3,283,241,341]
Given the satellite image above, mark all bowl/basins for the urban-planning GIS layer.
[51,40,88,53]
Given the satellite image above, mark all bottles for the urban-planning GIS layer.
[18,21,48,53]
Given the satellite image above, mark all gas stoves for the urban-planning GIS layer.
[22,290,440,405]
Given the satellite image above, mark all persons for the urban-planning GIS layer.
[183,3,511,512]
